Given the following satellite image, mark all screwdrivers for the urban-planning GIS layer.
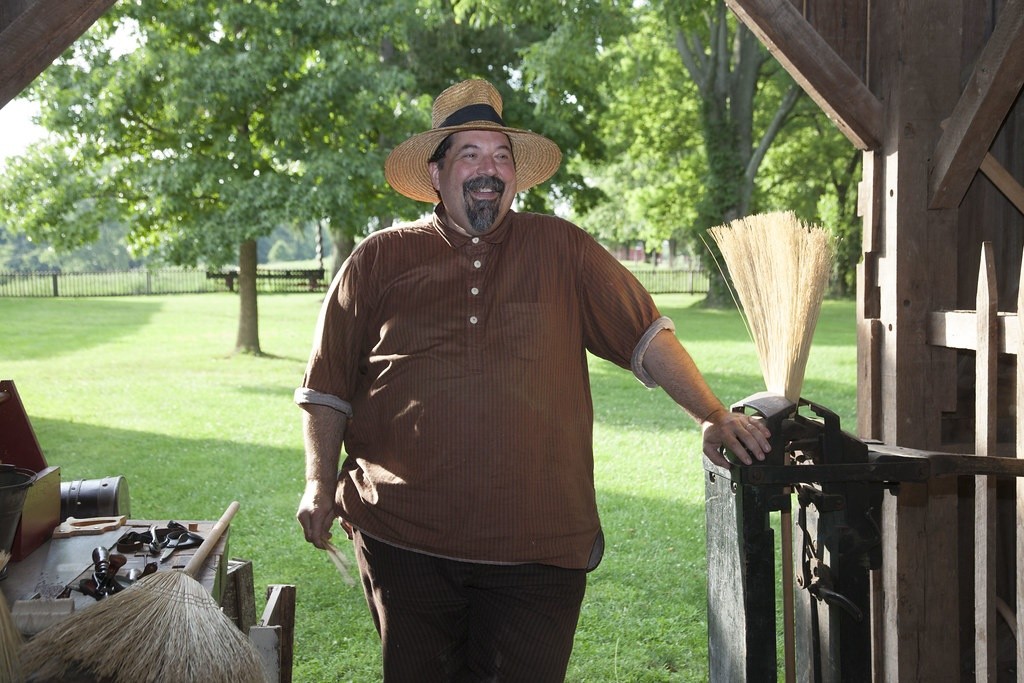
[93,547,110,597]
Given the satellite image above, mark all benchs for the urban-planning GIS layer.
[207,268,325,293]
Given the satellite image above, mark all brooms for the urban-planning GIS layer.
[692,207,831,683]
[21,500,270,682]
[0,548,21,683]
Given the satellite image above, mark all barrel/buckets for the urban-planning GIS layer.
[0,463,37,581]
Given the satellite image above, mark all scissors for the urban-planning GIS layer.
[159,530,204,564]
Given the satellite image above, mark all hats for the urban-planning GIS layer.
[384,78,564,204]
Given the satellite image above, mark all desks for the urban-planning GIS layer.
[0,519,230,640]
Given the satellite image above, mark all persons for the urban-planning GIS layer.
[297,129,771,683]
